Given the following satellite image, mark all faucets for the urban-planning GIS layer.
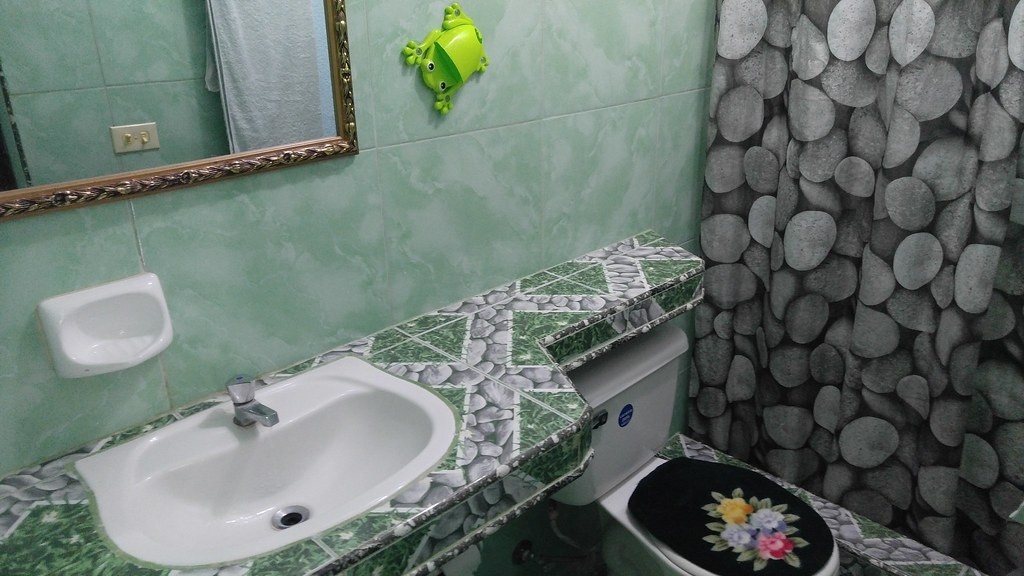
[226,374,279,427]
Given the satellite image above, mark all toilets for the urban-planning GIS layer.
[549,321,840,576]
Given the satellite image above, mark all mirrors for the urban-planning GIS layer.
[0,0,360,224]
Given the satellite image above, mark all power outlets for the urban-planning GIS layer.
[109,121,160,154]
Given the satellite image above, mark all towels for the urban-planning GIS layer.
[205,0,337,154]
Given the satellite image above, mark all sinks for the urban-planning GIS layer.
[67,353,461,571]
